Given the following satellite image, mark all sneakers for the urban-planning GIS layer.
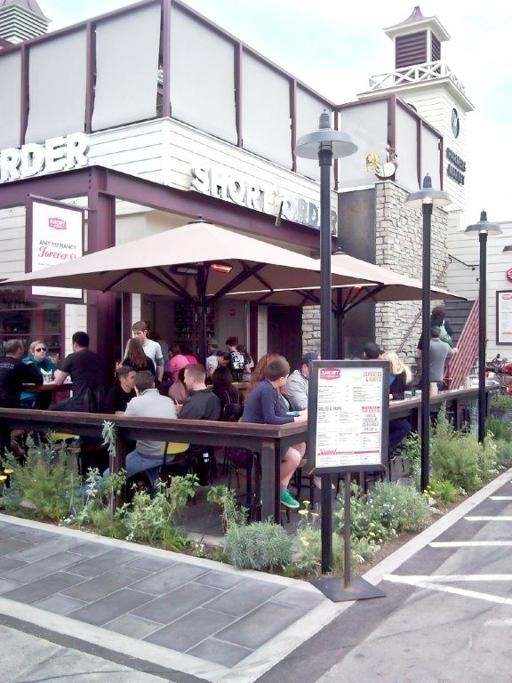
[280,489,301,508]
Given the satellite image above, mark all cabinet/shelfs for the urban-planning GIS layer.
[171,297,217,358]
[0,306,62,338]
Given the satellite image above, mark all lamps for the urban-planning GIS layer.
[170,264,199,275]
[207,261,233,272]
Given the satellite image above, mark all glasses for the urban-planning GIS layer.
[35,348,47,351]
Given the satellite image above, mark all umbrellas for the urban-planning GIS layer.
[205,247,468,361]
[1,213,385,373]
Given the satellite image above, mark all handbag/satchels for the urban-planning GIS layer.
[439,326,452,345]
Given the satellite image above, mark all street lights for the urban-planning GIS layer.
[292,108,359,576]
[402,173,454,495]
[464,209,503,450]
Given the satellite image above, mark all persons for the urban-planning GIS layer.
[414,306,454,386]
[167,364,221,478]
[168,354,198,402]
[223,335,245,381]
[54,331,120,415]
[281,353,335,491]
[241,356,307,509]
[426,327,458,390]
[18,339,61,413]
[101,364,136,412]
[121,338,156,381]
[387,419,410,455]
[207,339,219,353]
[236,344,254,380]
[1,338,43,408]
[363,343,381,360]
[101,370,177,506]
[116,321,164,382]
[377,351,413,401]
[209,366,244,419]
[206,350,231,377]
[243,352,294,415]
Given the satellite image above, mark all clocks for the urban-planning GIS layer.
[451,107,460,138]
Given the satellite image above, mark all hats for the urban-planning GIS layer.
[303,351,320,362]
[207,337,219,345]
